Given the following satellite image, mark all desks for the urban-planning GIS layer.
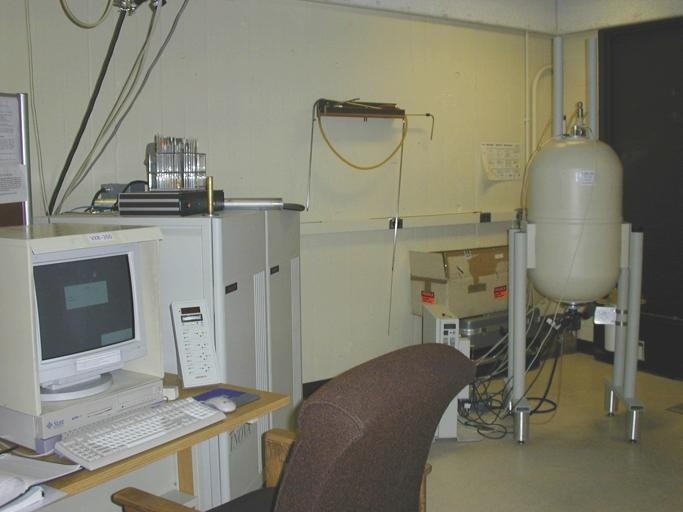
[2,372,291,509]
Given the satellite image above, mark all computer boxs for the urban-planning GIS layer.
[1,368,166,455]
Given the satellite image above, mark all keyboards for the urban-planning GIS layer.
[61,397,228,470]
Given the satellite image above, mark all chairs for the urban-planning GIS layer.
[109,342,477,511]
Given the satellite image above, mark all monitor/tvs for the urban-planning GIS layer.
[28,243,150,401]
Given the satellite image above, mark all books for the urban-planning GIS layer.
[0,451,83,509]
[0,485,47,512]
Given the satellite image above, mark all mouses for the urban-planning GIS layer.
[206,393,236,413]
